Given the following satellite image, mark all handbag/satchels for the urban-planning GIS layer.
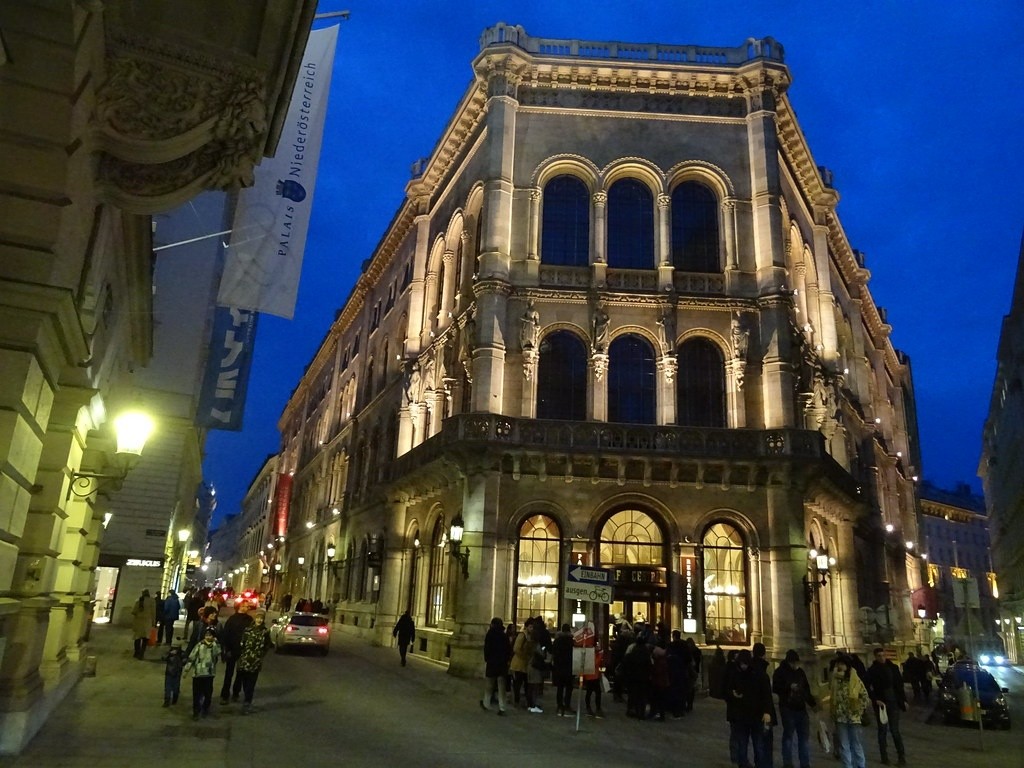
[507,633,526,656]
[879,702,888,724]
[149,627,157,643]
[530,651,546,670]
[817,712,832,753]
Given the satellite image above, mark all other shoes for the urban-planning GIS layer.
[595,709,605,719]
[202,711,208,718]
[193,712,199,720]
[586,710,593,716]
[557,708,562,717]
[401,659,406,667]
[563,708,576,717]
[133,650,144,660]
[219,698,229,705]
[480,700,488,711]
[241,700,257,715]
[497,710,505,715]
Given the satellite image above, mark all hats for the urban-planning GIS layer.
[786,649,799,662]
[255,608,266,616]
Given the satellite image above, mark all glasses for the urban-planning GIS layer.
[206,627,215,630]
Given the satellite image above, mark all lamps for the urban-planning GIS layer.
[263,565,272,579]
[297,554,308,579]
[326,542,344,577]
[183,548,199,564]
[910,603,926,636]
[803,537,829,608]
[1014,613,1024,630]
[65,408,155,501]
[274,559,287,579]
[239,565,247,575]
[1004,616,1012,629]
[167,528,191,554]
[994,616,1002,628]
[444,511,468,580]
[800,325,808,333]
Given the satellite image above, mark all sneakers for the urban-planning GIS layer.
[528,706,543,712]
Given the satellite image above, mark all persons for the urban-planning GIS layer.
[131,585,334,720]
[724,645,969,766]
[479,615,702,721]
[391,607,417,668]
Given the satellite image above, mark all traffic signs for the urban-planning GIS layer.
[564,565,615,604]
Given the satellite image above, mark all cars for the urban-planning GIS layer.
[977,651,1004,666]
[936,668,1011,730]
[234,590,261,608]
[952,660,979,667]
[207,586,229,600]
[270,611,330,656]
